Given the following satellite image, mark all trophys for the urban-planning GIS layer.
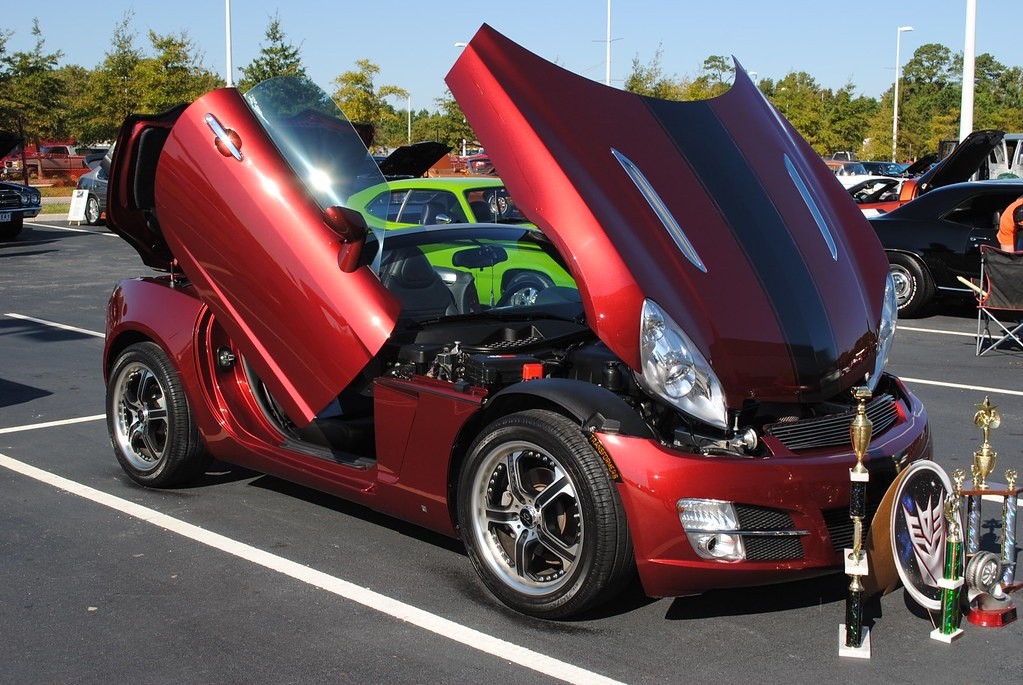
[943,470,966,539]
[973,395,1000,489]
[849,387,873,472]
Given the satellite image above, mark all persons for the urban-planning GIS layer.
[997,196,1023,251]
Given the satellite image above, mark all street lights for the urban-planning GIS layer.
[748,71,758,87]
[781,86,790,121]
[889,26,916,168]
[452,43,469,159]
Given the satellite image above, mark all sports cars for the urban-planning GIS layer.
[76,139,118,226]
[101,22,934,622]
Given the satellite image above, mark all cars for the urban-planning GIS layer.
[0,177,44,239]
[355,137,515,217]
[821,129,1023,218]
[347,174,578,308]
[865,179,1023,321]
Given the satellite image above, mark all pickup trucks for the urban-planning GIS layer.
[4,145,110,180]
[0,139,109,171]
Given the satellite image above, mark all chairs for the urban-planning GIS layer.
[420,202,446,225]
[970,244,1023,357]
[382,246,460,328]
[469,202,492,223]
